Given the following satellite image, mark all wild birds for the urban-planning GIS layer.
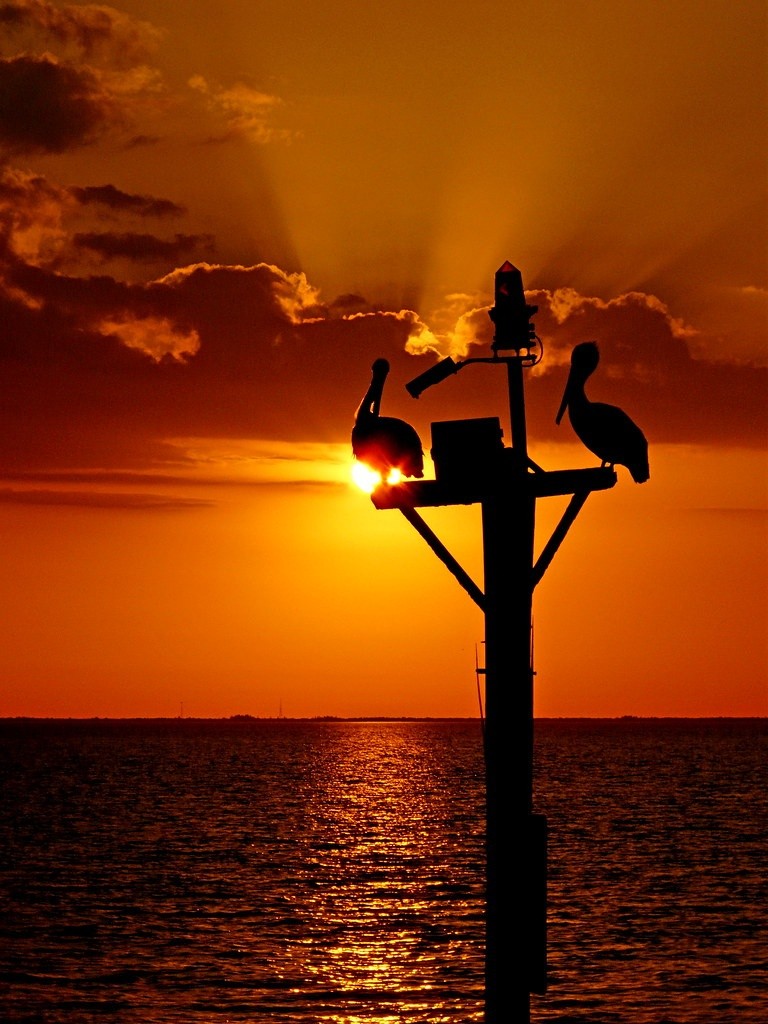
[351,359,424,489]
[555,342,650,486]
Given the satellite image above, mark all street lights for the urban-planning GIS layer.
[371,257,621,1023]
